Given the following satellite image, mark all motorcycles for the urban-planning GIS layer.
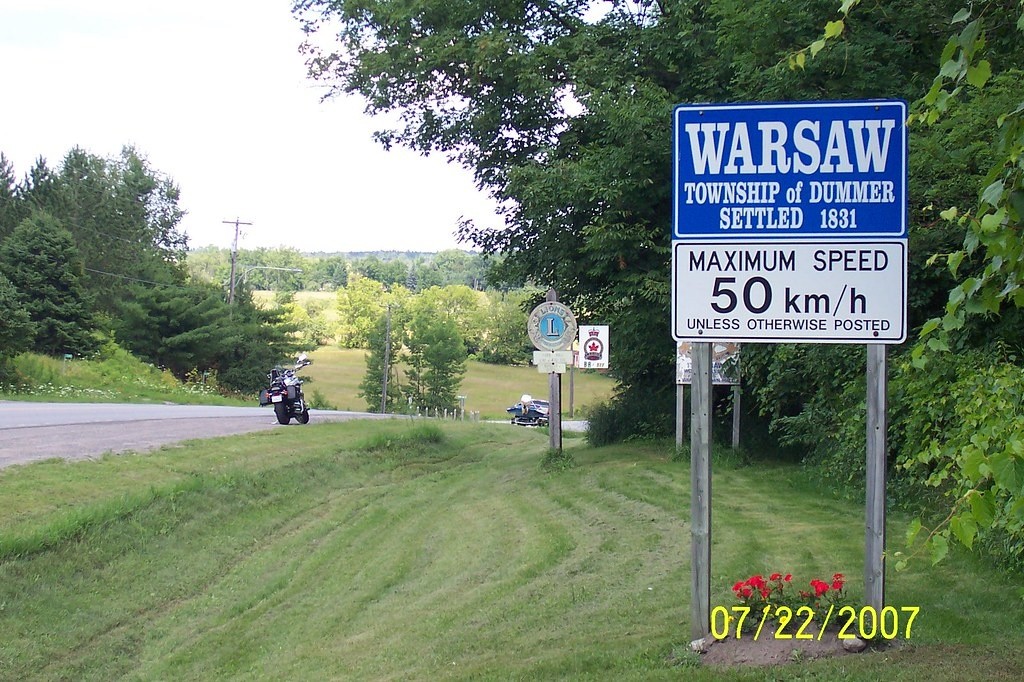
[260,355,311,424]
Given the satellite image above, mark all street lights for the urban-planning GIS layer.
[229,267,302,319]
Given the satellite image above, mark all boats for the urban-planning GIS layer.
[506,401,548,417]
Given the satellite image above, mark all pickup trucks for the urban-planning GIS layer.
[511,395,549,427]
